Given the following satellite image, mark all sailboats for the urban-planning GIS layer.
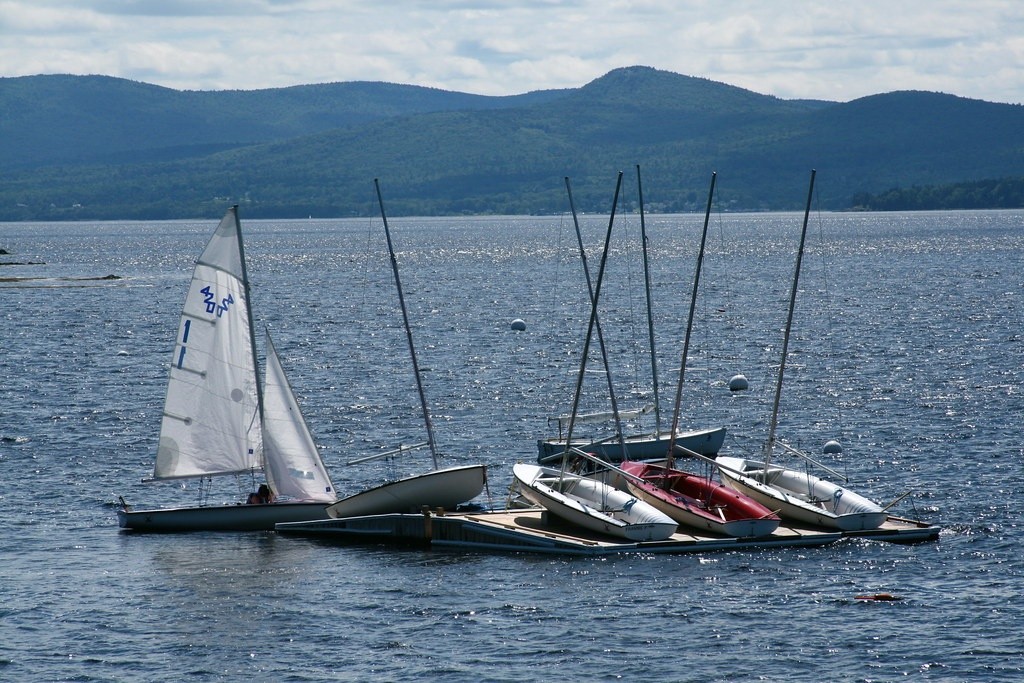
[323,174,490,517]
[509,159,892,543]
[119,204,343,532]
